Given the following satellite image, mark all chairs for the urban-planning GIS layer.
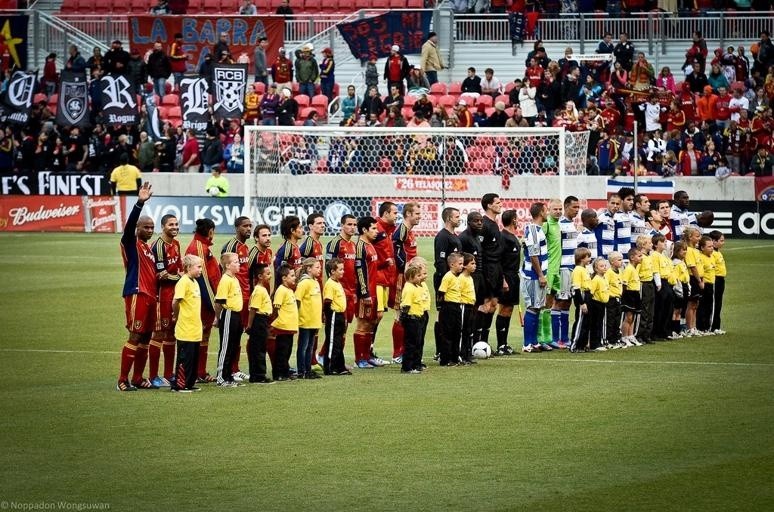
[34,81,743,176]
[60,0,423,31]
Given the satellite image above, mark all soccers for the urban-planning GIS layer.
[472,342,492,358]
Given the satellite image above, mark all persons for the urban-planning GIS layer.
[0,0,774,394]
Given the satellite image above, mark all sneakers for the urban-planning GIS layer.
[394,344,496,374]
[116,356,389,392]
[496,328,728,354]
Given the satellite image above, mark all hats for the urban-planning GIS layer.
[477,101,506,114]
